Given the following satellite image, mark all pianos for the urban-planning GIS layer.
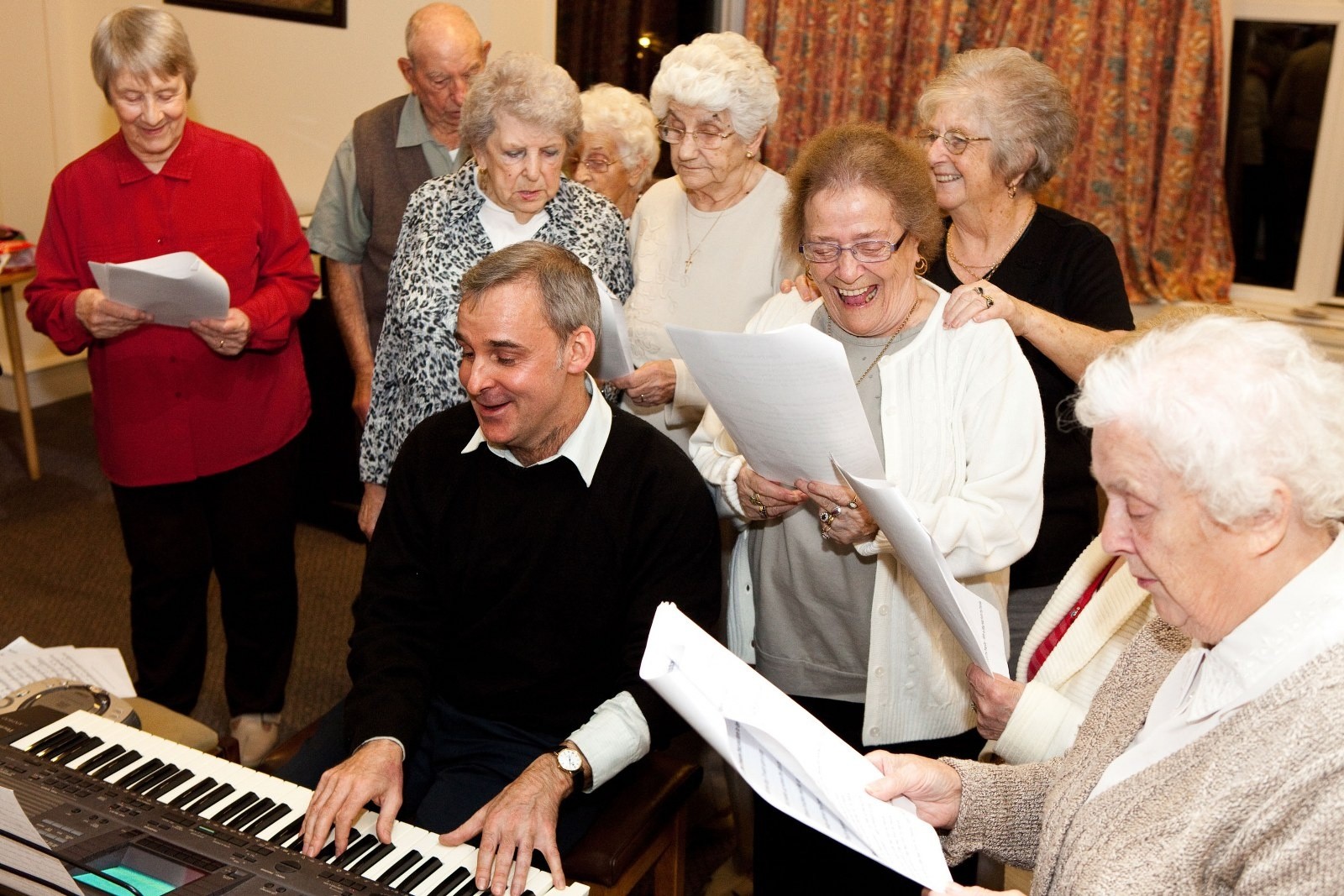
[0,708,611,896]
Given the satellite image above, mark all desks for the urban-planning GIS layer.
[0,271,42,480]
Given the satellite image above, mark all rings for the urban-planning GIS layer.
[827,515,834,524]
[831,506,841,518]
[750,493,761,506]
[848,494,858,509]
[822,532,830,540]
[969,703,977,714]
[758,506,768,519]
[826,527,831,533]
[821,525,827,532]
[982,295,994,308]
[220,341,224,348]
[640,394,645,404]
[820,513,829,522]
[974,287,984,297]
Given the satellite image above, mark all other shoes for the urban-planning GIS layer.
[230,712,282,768]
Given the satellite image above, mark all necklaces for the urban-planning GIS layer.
[828,294,918,388]
[1071,607,1082,617]
[946,195,1035,281]
[683,161,757,275]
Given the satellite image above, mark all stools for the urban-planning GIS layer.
[0,342,92,411]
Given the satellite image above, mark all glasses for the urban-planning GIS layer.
[797,226,911,263]
[567,152,634,175]
[655,116,737,150]
[915,128,1000,156]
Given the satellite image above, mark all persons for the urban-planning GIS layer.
[298,1,1344,896]
[22,5,320,768]
[300,240,723,896]
[863,313,1344,896]
[689,122,1045,896]
[358,50,634,542]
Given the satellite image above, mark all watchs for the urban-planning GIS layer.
[600,381,625,407]
[547,746,585,794]
